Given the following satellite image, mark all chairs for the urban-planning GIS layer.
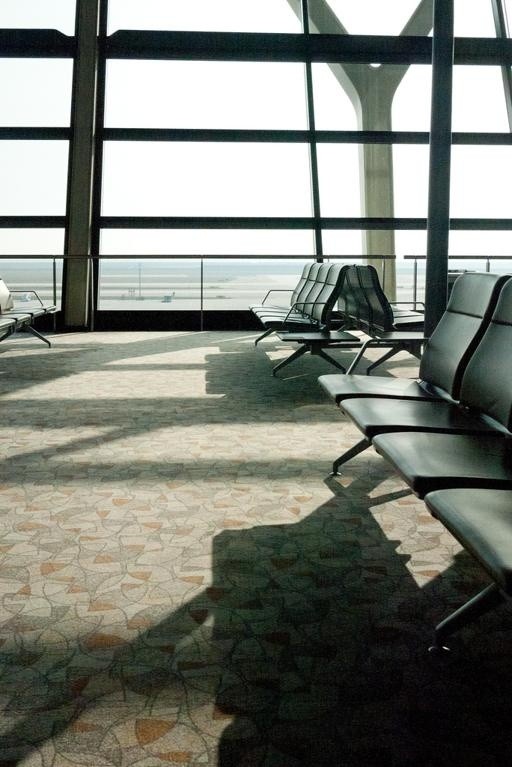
[0,290,57,348]
